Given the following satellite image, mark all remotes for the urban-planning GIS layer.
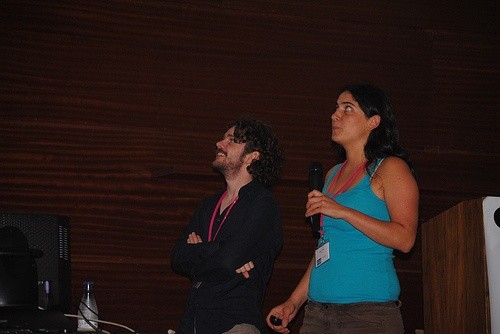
[270,315,292,328]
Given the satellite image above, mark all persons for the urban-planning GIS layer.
[266,85,419,334]
[171,119,284,334]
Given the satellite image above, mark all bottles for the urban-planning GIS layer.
[76,282,100,334]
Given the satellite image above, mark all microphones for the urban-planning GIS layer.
[307,160,322,239]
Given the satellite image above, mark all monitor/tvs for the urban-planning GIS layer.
[0,210,74,334]
